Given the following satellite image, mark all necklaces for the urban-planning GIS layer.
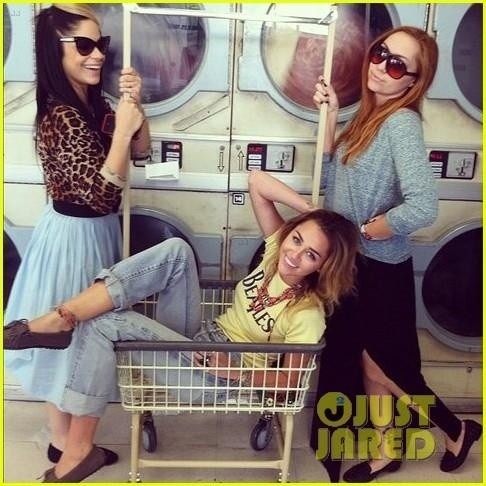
[249,278,299,313]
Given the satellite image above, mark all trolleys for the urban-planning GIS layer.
[113,278,327,484]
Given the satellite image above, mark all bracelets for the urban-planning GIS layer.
[236,368,254,385]
[357,219,377,243]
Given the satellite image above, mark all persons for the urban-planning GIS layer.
[5,4,150,466]
[5,204,360,481]
[313,26,483,482]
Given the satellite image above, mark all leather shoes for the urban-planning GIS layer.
[49,442,118,465]
[440,419,483,471]
[343,453,402,482]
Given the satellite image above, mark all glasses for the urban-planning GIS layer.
[369,45,418,79]
[59,35,111,57]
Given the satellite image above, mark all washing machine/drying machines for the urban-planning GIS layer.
[85,4,234,133]
[106,131,228,408]
[228,140,327,407]
[4,1,46,126]
[420,3,483,146]
[404,148,483,415]
[233,2,427,141]
[4,130,47,402]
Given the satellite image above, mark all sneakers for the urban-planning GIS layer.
[4,319,73,350]
[37,444,106,483]
